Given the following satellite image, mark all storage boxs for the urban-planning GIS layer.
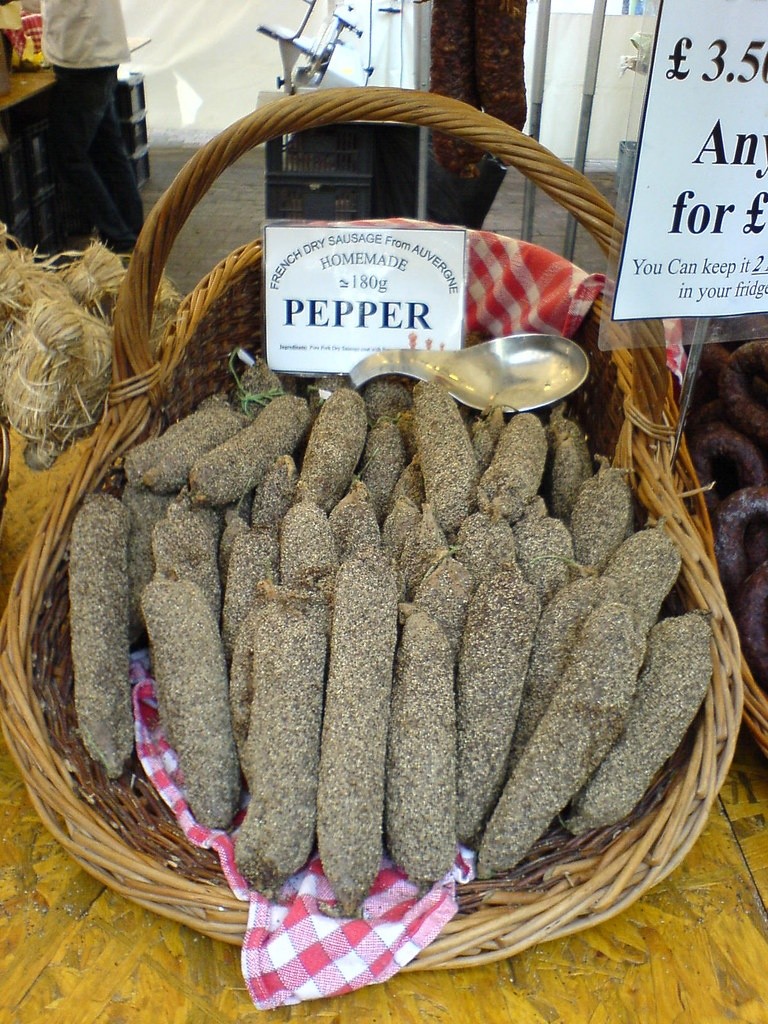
[264,123,375,182]
[110,70,151,188]
[0,109,78,264]
[265,178,376,224]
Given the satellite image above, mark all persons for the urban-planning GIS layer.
[40,0,144,251]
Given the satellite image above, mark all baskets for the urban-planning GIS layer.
[664,368,768,755]
[0,84,742,973]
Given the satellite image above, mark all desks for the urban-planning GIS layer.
[0,36,151,109]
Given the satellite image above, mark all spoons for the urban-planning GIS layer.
[346,333,590,414]
